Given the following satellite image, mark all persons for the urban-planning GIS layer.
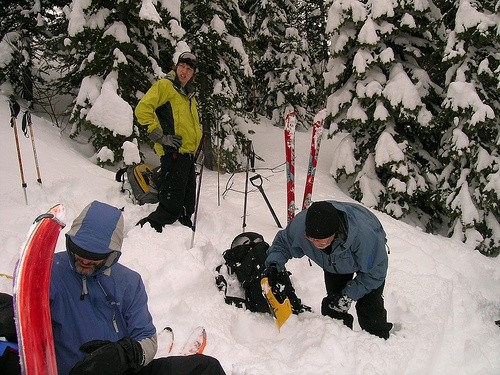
[136,52,206,233]
[264,201,393,341]
[0,200,226,375]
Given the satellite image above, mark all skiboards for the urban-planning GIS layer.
[284,109,327,225]
[151,327,207,359]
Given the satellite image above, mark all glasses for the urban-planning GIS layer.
[183,54,196,61]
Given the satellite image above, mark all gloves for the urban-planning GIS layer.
[157,134,182,152]
[329,292,352,314]
[262,263,278,286]
[69,336,143,375]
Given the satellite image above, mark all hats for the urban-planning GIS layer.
[305,201,339,239]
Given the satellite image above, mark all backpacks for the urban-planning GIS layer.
[224,239,304,315]
[127,162,159,206]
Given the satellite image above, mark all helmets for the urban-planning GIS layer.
[176,52,198,70]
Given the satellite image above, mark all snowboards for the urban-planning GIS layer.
[12,203,66,375]
